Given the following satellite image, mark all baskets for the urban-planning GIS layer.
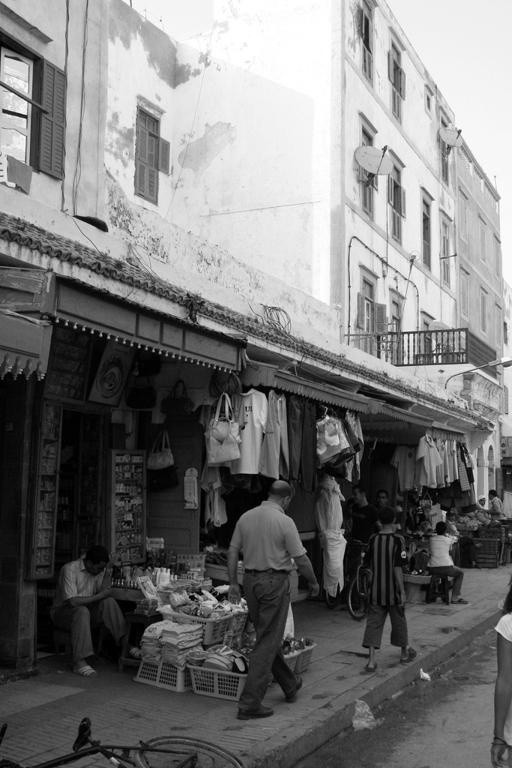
[172,608,247,645]
[279,643,317,673]
[132,658,249,701]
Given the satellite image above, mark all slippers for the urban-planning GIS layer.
[401,648,416,663]
[452,598,467,604]
[366,662,377,673]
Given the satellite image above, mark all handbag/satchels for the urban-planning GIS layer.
[125,367,192,415]
[206,392,241,465]
[147,430,179,491]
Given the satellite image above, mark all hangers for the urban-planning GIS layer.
[316,405,329,422]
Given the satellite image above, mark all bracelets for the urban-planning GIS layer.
[491,736,508,746]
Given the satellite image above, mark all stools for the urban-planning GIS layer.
[119,611,162,673]
[430,573,457,604]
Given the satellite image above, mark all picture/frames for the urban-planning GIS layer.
[84,337,143,408]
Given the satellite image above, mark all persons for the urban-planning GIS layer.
[425,519,469,606]
[49,546,143,681]
[489,586,512,768]
[228,478,321,720]
[477,490,505,521]
[347,484,417,671]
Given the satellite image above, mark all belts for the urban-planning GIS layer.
[245,569,288,574]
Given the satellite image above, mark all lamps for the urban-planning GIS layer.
[444,354,511,392]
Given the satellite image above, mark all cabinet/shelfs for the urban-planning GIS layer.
[23,403,63,582]
[108,452,146,565]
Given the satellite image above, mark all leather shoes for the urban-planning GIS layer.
[285,676,302,704]
[237,704,274,720]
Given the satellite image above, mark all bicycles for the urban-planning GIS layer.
[347,538,377,618]
[0,712,246,766]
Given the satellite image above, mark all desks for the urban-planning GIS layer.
[202,561,243,591]
[89,578,212,674]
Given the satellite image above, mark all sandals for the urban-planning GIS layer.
[72,666,97,678]
[129,647,144,658]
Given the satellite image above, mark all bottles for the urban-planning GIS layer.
[111,567,176,591]
